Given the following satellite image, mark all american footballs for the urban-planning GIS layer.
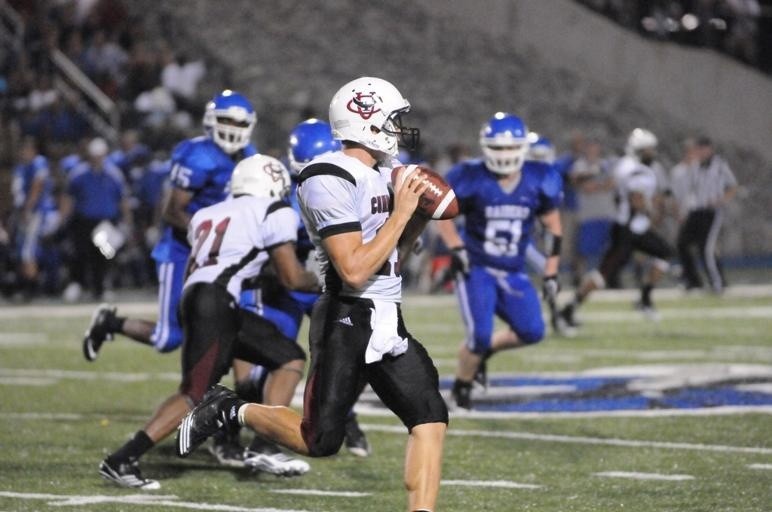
[391,166,459,219]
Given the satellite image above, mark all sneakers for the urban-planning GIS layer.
[100,454,162,491]
[449,349,490,416]
[635,299,662,324]
[548,299,578,340]
[83,301,119,361]
[175,386,377,477]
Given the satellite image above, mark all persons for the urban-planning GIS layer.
[81,88,263,470]
[100,154,321,493]
[174,74,448,512]
[244,116,373,458]
[438,111,564,412]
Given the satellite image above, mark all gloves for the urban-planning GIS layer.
[540,279,563,304]
[446,245,471,282]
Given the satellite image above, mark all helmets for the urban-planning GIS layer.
[526,133,556,165]
[328,75,411,158]
[287,117,341,172]
[203,91,260,154]
[478,113,528,172]
[623,128,657,154]
[231,154,291,204]
